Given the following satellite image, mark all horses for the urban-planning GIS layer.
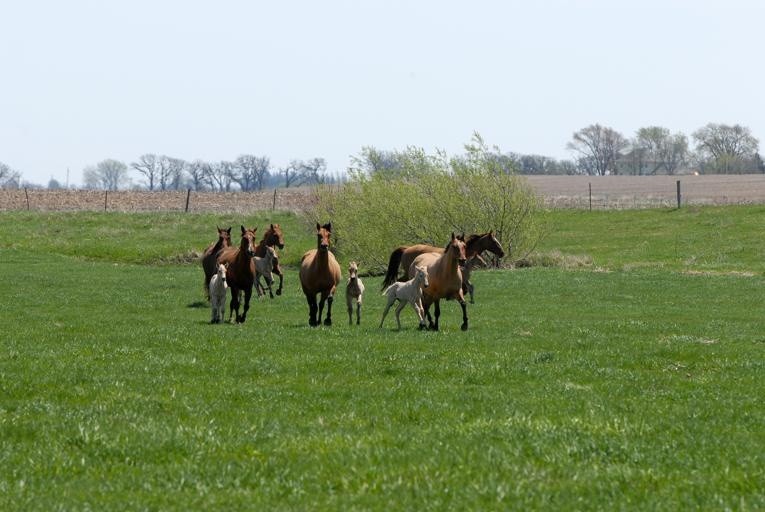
[378,229,505,331]
[299,220,342,328]
[345,260,365,325]
[201,223,285,324]
